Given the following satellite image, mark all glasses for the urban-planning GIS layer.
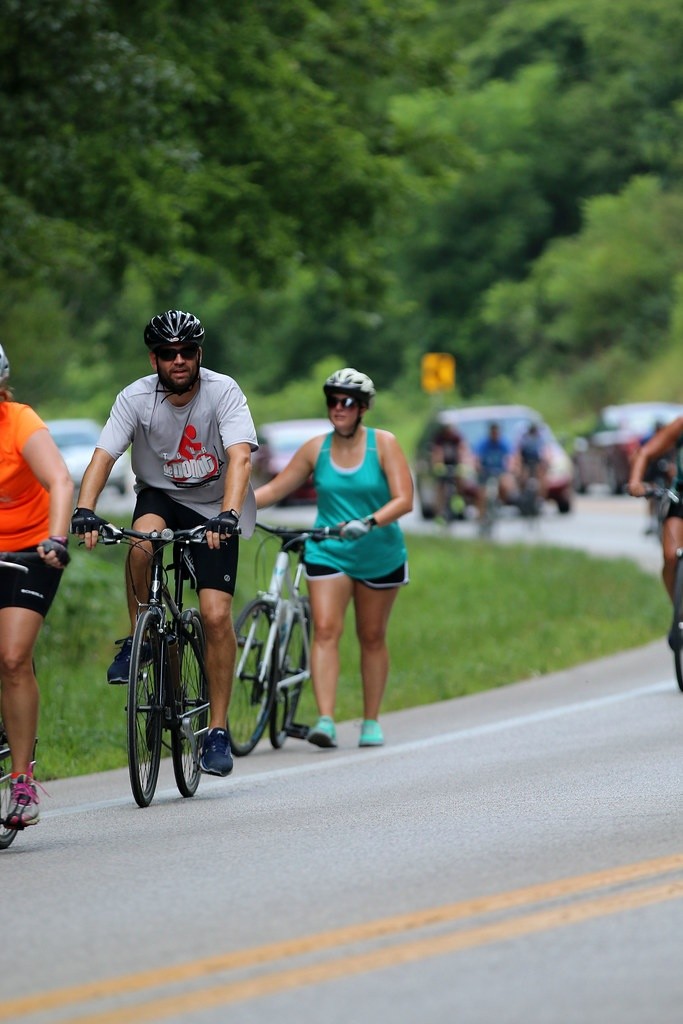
[327,395,355,408]
[157,344,199,361]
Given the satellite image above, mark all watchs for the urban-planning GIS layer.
[367,515,379,529]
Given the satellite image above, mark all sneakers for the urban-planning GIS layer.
[358,720,384,745]
[308,719,339,747]
[5,761,50,826]
[107,636,153,684]
[200,728,233,777]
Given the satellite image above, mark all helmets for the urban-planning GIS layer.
[0,344,7,377]
[325,368,376,399]
[144,310,206,350]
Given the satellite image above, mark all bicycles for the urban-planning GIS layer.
[627,489,683,696]
[226,520,346,756]
[434,453,544,528]
[0,550,49,853]
[78,520,241,809]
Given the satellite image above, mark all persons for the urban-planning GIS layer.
[253,367,414,745]
[71,309,260,776]
[626,409,683,615]
[0,343,75,828]
[431,421,552,526]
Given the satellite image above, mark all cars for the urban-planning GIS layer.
[253,419,336,507]
[414,405,574,522]
[43,417,127,497]
[571,403,683,494]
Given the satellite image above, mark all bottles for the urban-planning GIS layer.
[164,621,180,688]
[276,600,292,647]
